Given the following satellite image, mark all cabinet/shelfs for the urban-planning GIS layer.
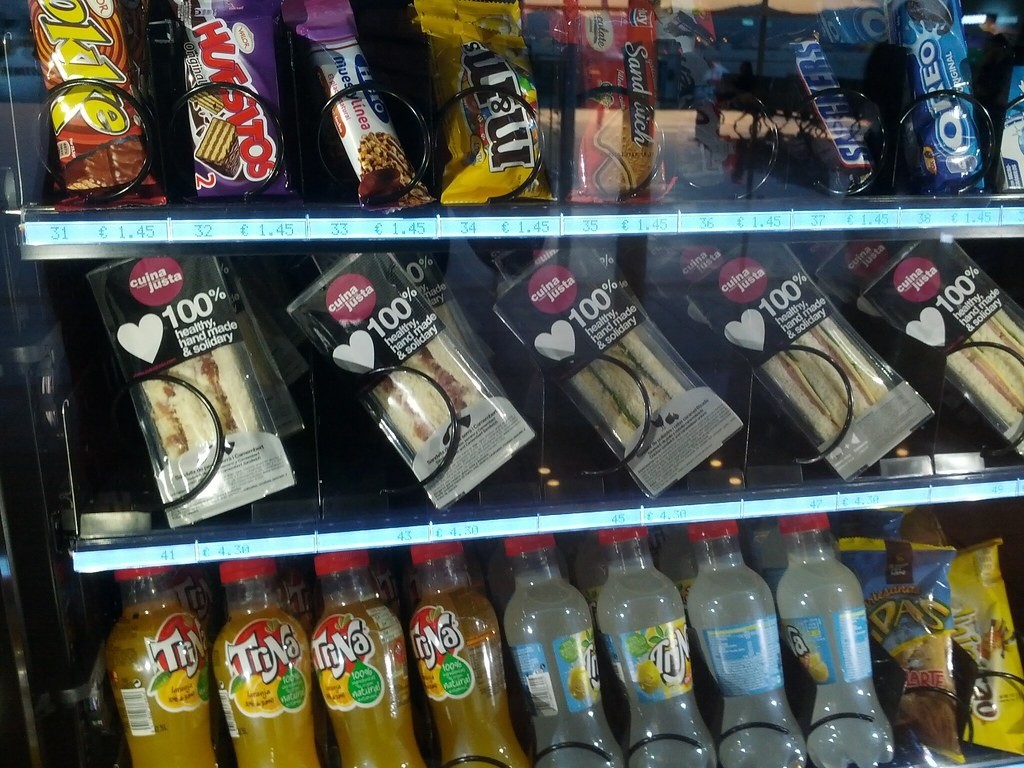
[0,0,1024,768]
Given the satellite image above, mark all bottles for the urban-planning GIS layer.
[106,568,218,767]
[409,541,529,768]
[504,534,624,767]
[211,561,323,768]
[775,512,895,768]
[686,520,808,768]
[597,527,719,767]
[310,551,424,767]
